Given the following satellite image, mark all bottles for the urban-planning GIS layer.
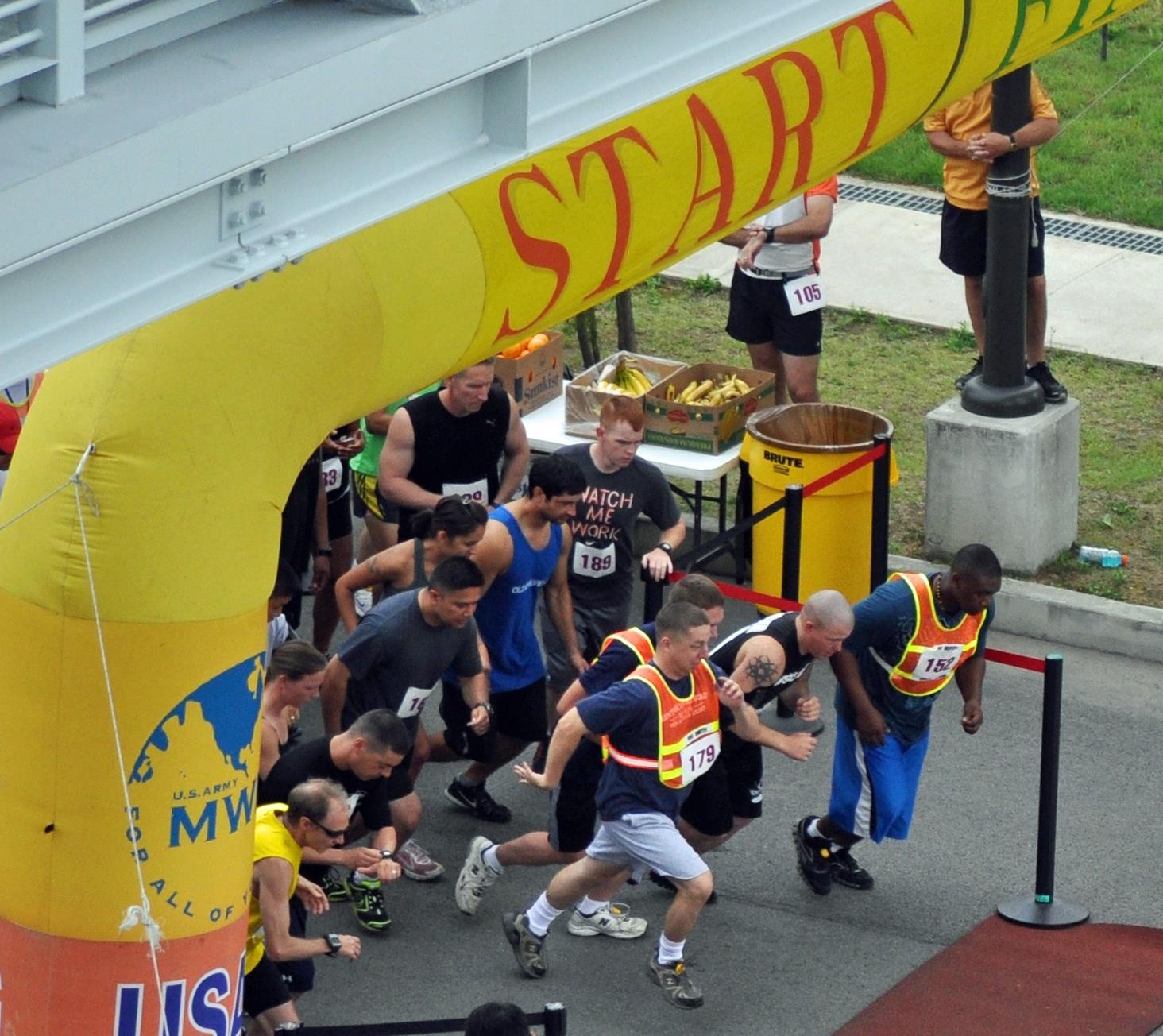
[1081,544,1127,569]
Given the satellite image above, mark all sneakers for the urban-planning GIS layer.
[955,356,985,389]
[1027,361,1067,401]
[393,839,445,880]
[501,908,550,979]
[647,952,704,1010]
[443,778,509,821]
[567,902,648,940]
[792,815,832,895]
[314,866,346,902]
[344,870,391,932]
[829,849,875,890]
[455,835,503,915]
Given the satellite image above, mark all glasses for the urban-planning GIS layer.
[308,818,347,838]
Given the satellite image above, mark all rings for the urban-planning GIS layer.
[290,715,295,720]
[391,870,397,874]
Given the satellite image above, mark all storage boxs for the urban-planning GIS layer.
[645,362,776,455]
[566,350,687,441]
[494,327,565,418]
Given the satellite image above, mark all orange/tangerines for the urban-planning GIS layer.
[495,334,550,360]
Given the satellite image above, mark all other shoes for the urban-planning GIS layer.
[353,589,372,616]
[648,869,719,903]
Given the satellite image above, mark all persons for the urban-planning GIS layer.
[921,59,1071,405]
[717,173,840,410]
[245,351,854,1034]
[790,545,1009,892]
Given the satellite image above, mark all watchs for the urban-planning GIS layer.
[656,542,672,557]
[1008,134,1018,152]
[474,701,495,715]
[321,933,342,960]
[379,849,395,860]
[762,225,776,244]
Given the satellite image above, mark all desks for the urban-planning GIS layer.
[514,381,738,580]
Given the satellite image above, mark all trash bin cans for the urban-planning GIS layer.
[739,403,899,616]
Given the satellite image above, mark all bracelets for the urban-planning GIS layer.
[490,501,501,510]
[316,546,334,560]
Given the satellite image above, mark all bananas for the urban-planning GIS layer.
[598,356,653,399]
[666,374,751,407]
